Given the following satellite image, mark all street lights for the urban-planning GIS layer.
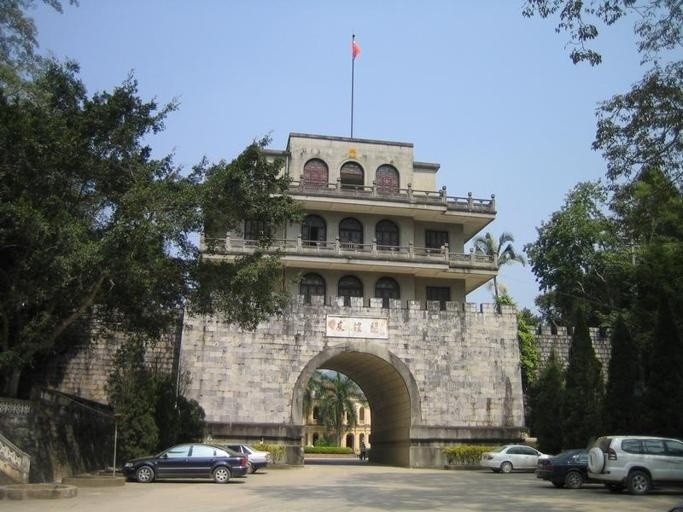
[282,150,290,180]
[112,412,121,479]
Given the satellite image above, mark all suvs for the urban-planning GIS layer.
[586,435,682,495]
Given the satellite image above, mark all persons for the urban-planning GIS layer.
[359,442,367,460]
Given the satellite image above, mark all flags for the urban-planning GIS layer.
[353,40,361,59]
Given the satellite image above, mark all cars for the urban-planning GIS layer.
[208,443,271,474]
[479,444,554,473]
[534,448,604,489]
[122,442,248,483]
